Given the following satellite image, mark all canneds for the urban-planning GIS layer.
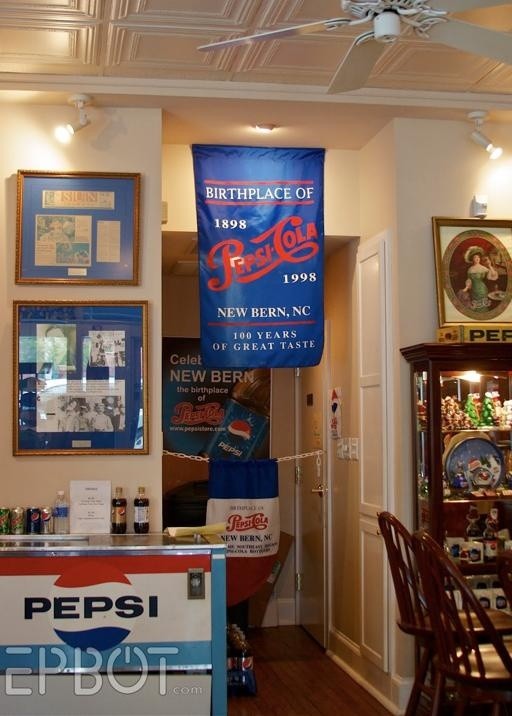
[11,507,25,534]
[40,506,54,534]
[26,508,40,534]
[0,507,10,535]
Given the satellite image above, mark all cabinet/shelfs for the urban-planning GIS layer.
[400,343,512,615]
[15,168,141,286]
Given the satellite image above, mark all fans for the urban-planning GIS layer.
[197,0,512,95]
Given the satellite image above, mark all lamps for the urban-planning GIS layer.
[55,94,92,143]
[469,110,503,161]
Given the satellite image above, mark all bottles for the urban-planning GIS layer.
[53,488,68,534]
[111,485,127,534]
[199,365,269,460]
[133,485,149,533]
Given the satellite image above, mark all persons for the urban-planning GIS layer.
[460,244,497,313]
[57,396,125,432]
[89,332,126,367]
[38,324,78,376]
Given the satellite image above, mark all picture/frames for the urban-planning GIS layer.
[13,300,149,456]
[432,216,512,328]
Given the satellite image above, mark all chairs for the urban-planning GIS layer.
[378,511,512,716]
